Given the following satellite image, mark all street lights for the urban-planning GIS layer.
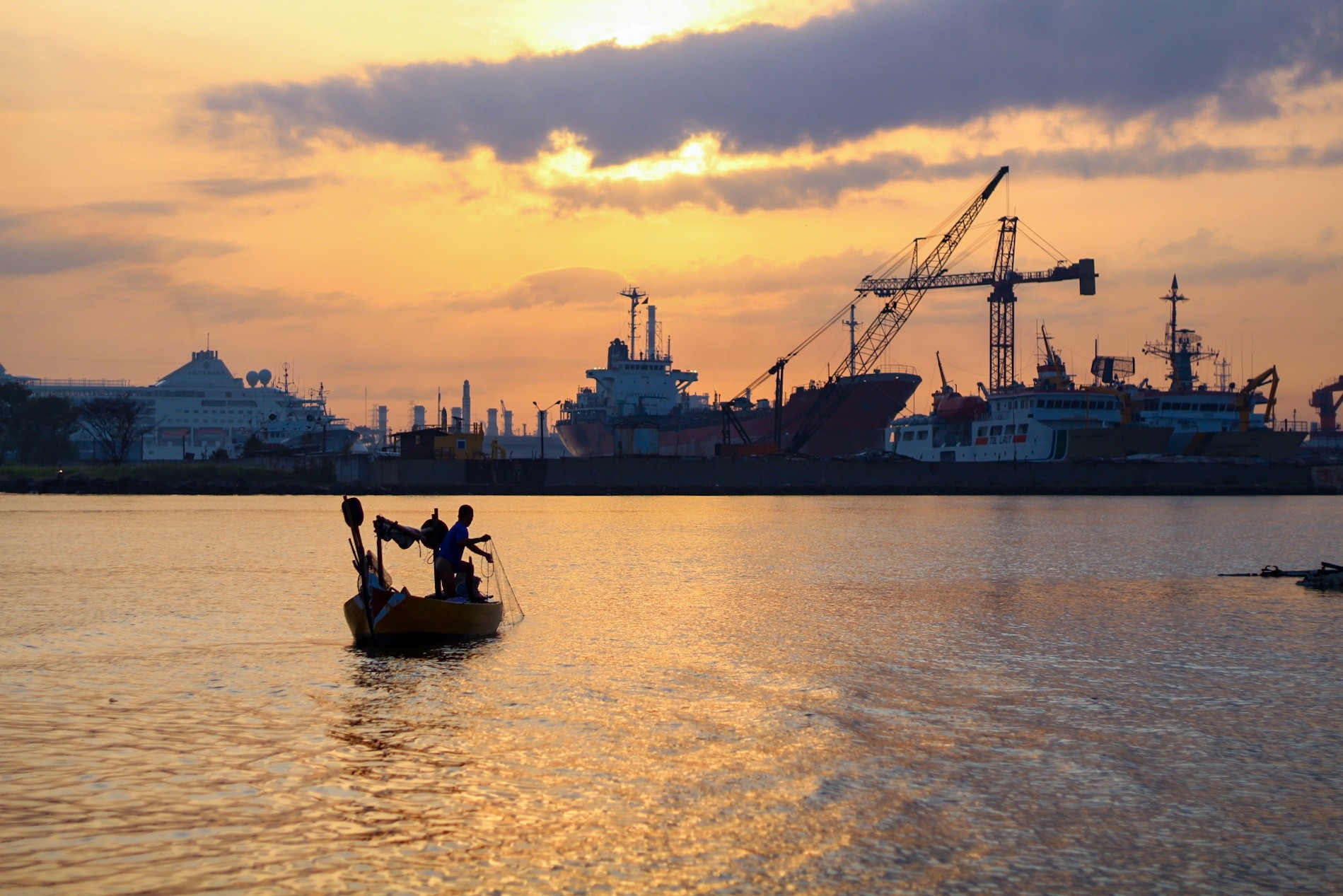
[533,400,562,457]
[183,431,190,460]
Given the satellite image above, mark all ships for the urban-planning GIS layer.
[555,284,923,455]
[0,331,368,459]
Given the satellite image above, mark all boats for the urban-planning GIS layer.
[219,361,361,455]
[343,493,505,646]
[885,267,1309,462]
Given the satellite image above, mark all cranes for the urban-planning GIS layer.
[856,216,1100,392]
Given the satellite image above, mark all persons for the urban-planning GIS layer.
[425,505,494,603]
